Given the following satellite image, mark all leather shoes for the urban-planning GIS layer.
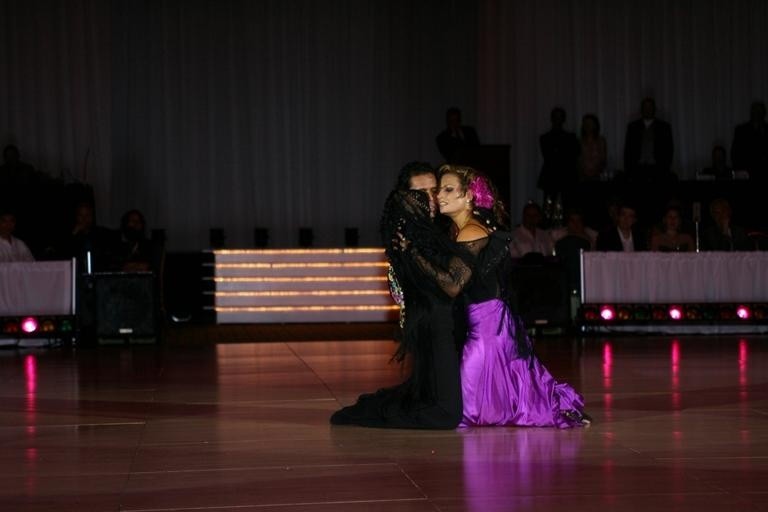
[329,392,387,427]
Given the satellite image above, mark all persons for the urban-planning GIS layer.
[391,165,590,428]
[103,207,154,272]
[1,206,36,263]
[436,107,485,169]
[326,159,510,432]
[62,197,102,272]
